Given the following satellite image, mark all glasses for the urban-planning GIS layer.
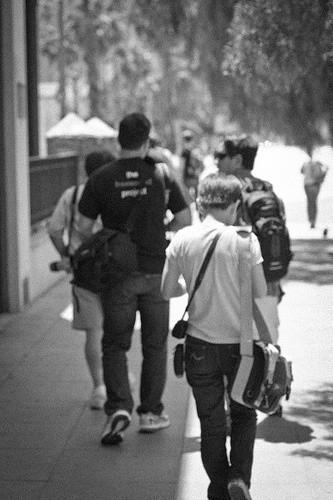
[213,151,227,160]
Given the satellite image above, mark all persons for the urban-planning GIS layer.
[176,127,202,203]
[301,149,327,228]
[146,133,177,176]
[48,149,120,412]
[73,113,193,448]
[214,134,286,345]
[158,170,269,500]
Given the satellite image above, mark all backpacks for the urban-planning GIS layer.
[238,178,294,282]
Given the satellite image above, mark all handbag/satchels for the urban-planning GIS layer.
[230,224,293,420]
[69,158,166,294]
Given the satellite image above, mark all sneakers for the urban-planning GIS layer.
[90,390,107,410]
[136,410,171,434]
[100,409,132,447]
